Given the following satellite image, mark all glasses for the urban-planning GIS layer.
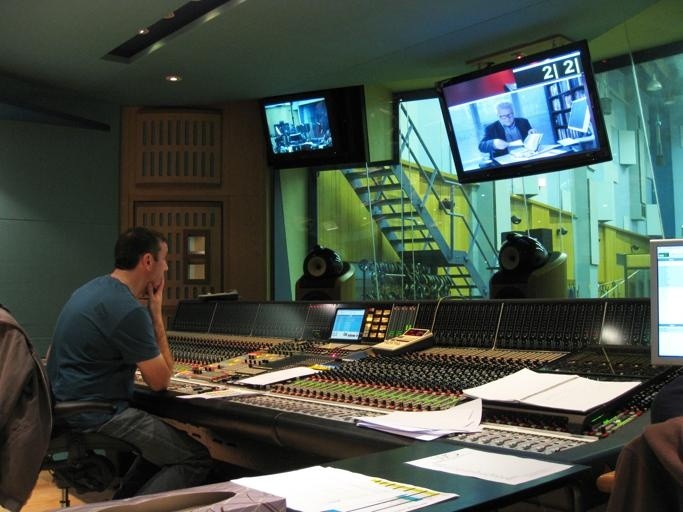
[500,113,513,119]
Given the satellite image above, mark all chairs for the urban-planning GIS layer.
[489,251,569,297]
[45,402,142,507]
[293,262,356,301]
[599,381,683,512]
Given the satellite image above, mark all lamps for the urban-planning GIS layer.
[645,71,677,108]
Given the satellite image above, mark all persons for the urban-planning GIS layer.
[39,223,217,497]
[476,103,533,161]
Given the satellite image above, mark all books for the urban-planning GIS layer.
[457,366,642,414]
[548,74,592,141]
[508,133,543,159]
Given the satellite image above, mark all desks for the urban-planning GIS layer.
[328,442,589,512]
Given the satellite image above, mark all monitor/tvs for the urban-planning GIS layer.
[328,308,366,343]
[649,238,683,366]
[258,85,370,169]
[435,39,613,183]
